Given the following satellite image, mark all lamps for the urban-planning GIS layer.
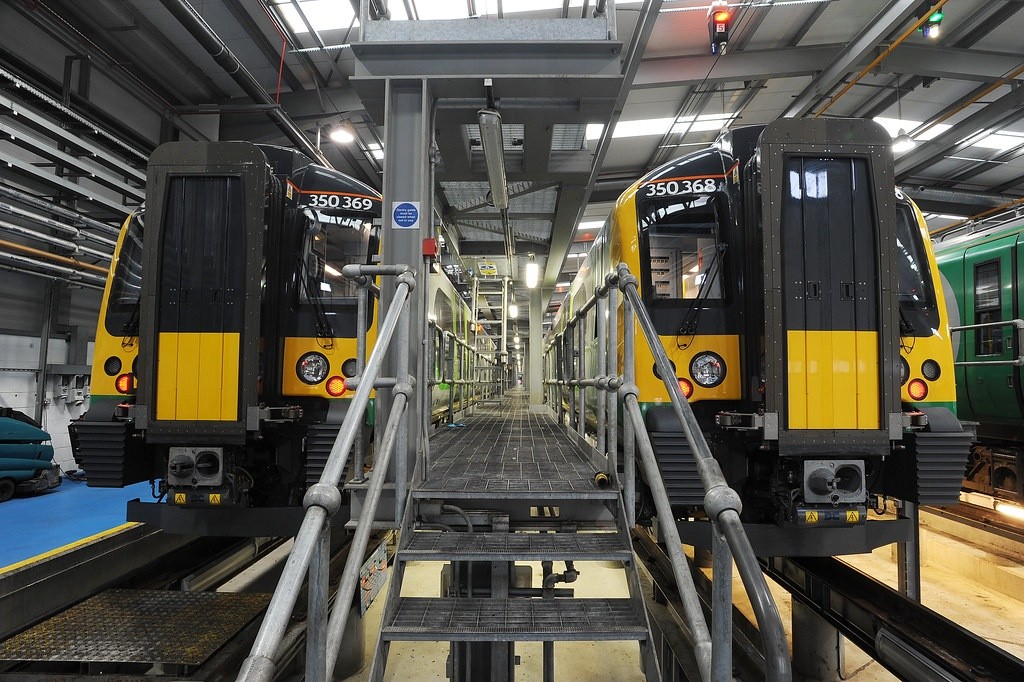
[331,118,354,143]
[891,72,916,152]
[477,108,509,210]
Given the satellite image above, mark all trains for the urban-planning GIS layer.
[68,140,498,538]
[928,198,1024,505]
[540,117,973,558]
